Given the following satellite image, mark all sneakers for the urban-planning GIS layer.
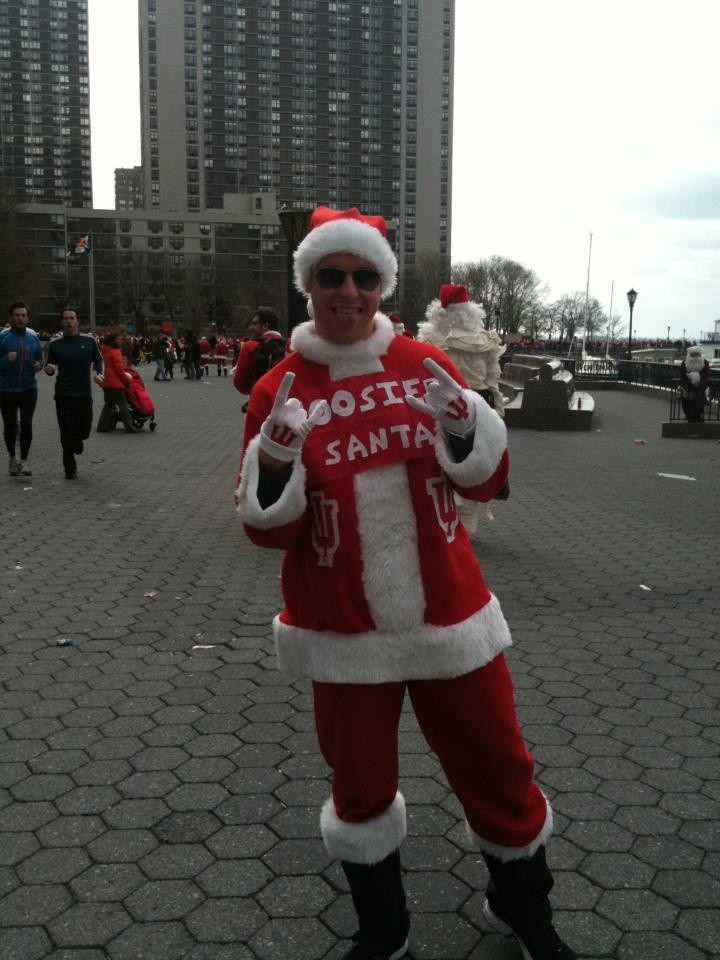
[18,459,33,476]
[7,457,20,475]
[64,466,77,479]
[73,438,84,454]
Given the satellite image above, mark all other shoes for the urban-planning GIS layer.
[126,426,144,433]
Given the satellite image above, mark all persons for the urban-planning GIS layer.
[505,335,696,355]
[4,322,253,383]
[95,331,143,434]
[414,285,507,540]
[233,203,580,958]
[2,300,47,478]
[388,311,414,340]
[231,306,290,410]
[43,307,106,480]
[680,346,710,423]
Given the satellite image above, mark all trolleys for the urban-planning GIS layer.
[125,368,156,431]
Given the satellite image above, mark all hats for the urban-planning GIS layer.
[289,206,399,317]
[687,346,703,354]
[435,285,471,316]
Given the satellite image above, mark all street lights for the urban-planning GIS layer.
[627,288,638,360]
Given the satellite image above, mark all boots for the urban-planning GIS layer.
[332,854,411,960]
[153,365,229,380]
[479,846,580,960]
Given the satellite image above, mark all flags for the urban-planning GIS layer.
[74,230,91,257]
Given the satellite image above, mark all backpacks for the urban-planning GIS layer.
[250,336,288,376]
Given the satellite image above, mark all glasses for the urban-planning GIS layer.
[312,264,381,292]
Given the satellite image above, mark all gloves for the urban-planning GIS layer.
[402,356,477,437]
[257,369,327,464]
